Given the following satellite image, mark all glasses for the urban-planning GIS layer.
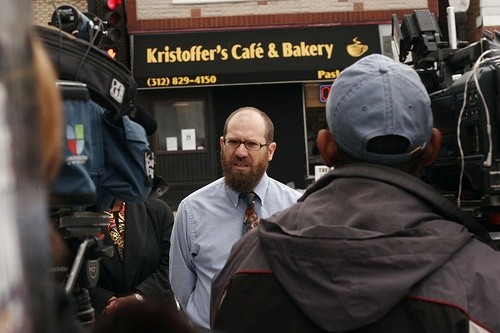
[223,137,270,151]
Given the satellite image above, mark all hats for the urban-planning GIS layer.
[327,54,434,163]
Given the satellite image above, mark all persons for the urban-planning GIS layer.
[166,104,310,333]
[50,97,157,219]
[85,195,182,333]
[206,51,499,331]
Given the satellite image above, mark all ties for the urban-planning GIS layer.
[242,192,259,236]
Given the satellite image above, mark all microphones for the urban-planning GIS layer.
[129,104,157,136]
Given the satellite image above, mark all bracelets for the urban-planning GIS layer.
[134,292,147,306]
